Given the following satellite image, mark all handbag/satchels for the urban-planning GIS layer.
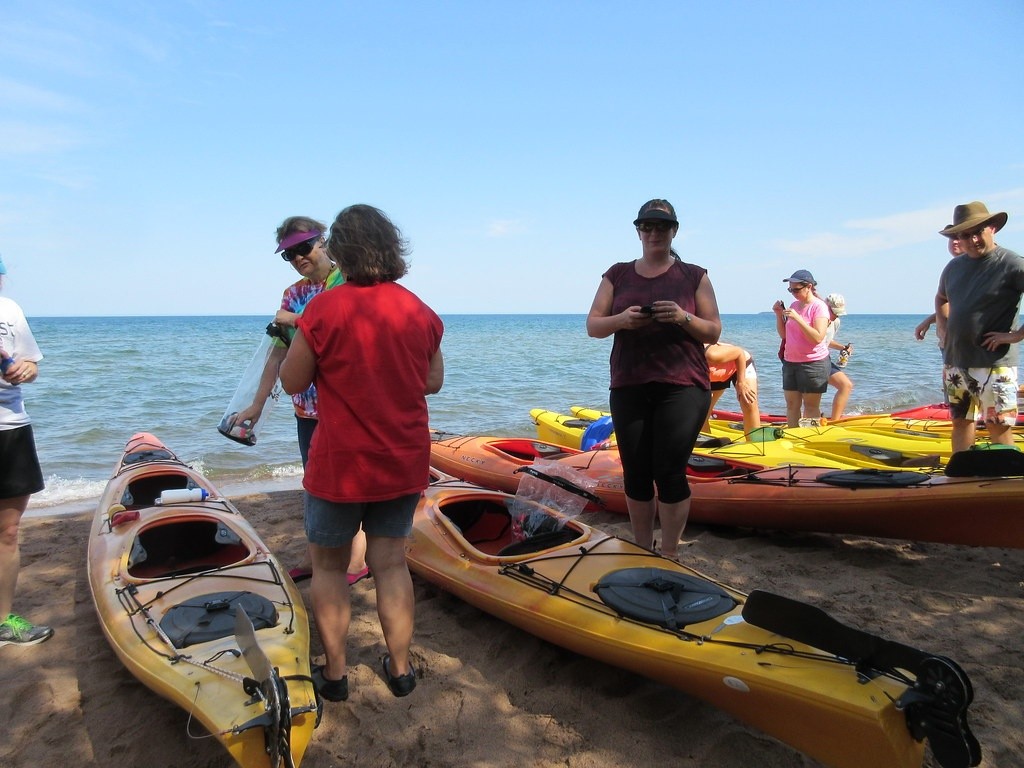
[778,339,786,359]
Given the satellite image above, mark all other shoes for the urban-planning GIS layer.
[381,654,416,696]
[312,664,349,701]
[346,566,369,585]
[287,567,312,583]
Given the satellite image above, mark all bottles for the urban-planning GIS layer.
[969,443,1021,453]
[161,488,209,504]
[834,342,854,368]
[749,428,784,441]
[728,424,744,430]
[797,417,828,427]
[709,414,717,419]
[591,441,609,450]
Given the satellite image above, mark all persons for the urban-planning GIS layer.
[914,200,1024,454]
[586,198,722,562]
[700,341,760,443]
[274,204,444,702]
[772,270,833,427]
[238,215,370,587]
[823,294,853,421]
[1,261,55,646]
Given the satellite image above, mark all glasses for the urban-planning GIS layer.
[281,237,320,261]
[959,223,989,239]
[637,220,675,233]
[788,285,808,293]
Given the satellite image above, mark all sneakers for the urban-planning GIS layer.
[0,614,53,647]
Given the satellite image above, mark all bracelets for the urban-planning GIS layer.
[678,312,691,326]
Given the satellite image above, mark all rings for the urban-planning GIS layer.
[993,340,995,343]
[22,372,27,376]
[669,312,671,317]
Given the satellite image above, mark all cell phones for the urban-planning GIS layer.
[639,305,655,315]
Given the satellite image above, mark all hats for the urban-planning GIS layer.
[783,270,814,286]
[633,209,679,226]
[939,201,1008,239]
[824,293,848,316]
[274,228,321,254]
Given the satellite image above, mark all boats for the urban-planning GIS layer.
[404,462,982,767]
[86,430,323,768]
[428,429,1023,550]
[529,386,1024,472]
